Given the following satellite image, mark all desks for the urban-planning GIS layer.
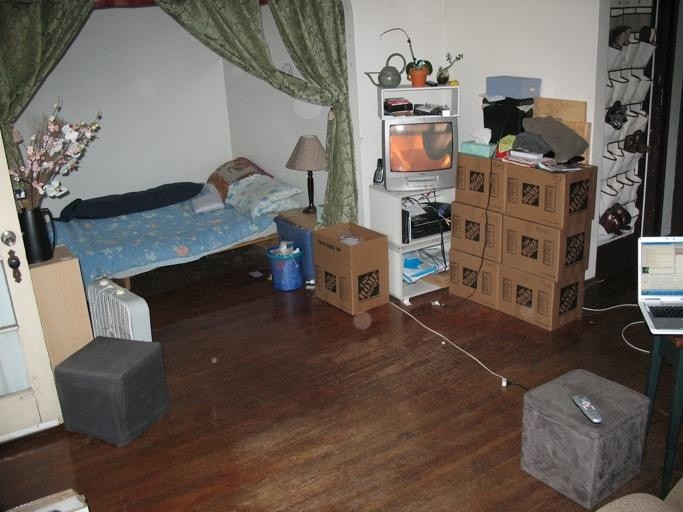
[647,333,682,500]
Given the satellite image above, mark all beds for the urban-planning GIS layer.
[45,155,309,293]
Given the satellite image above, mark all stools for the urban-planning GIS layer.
[53,336,168,449]
[518,365,651,508]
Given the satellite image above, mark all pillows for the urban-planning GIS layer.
[207,158,275,203]
[226,169,302,219]
[266,197,304,214]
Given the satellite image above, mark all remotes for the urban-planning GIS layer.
[571,394,602,424]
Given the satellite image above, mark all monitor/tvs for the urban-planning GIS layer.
[381,117,458,192]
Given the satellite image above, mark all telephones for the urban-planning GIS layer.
[373,159,385,186]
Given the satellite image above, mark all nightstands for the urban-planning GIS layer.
[275,205,324,282]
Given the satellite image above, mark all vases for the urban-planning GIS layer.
[17,207,58,263]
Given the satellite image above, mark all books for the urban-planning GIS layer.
[500,150,556,169]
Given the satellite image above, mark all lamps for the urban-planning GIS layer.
[285,133,332,217]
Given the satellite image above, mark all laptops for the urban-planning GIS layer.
[637,237,683,335]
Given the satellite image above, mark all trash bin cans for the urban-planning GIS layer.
[266,245,304,291]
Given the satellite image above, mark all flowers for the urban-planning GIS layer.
[9,96,108,212]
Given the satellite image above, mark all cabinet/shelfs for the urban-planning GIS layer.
[376,84,460,121]
[30,246,95,370]
[383,244,448,305]
[368,186,453,245]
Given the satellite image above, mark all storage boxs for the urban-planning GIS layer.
[316,218,389,317]
[441,147,598,334]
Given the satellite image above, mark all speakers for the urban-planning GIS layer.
[482,97,533,144]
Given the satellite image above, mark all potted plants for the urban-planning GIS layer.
[377,27,433,87]
[435,52,464,84]
